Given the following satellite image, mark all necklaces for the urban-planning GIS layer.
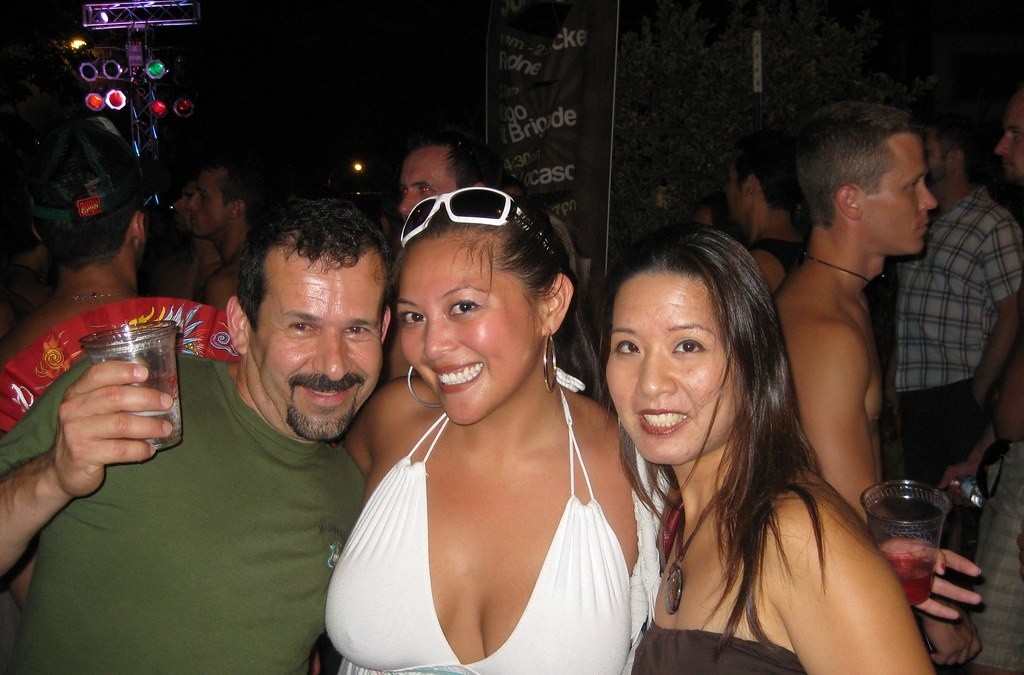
[663,485,725,615]
[52,291,131,301]
[804,253,870,284]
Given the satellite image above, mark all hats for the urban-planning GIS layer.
[25,115,142,219]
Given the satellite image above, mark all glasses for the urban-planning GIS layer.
[400,187,557,259]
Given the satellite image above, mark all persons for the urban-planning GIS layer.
[324,184,985,675]
[595,219,936,675]
[1,119,527,384]
[1,200,393,675]
[691,83,1024,675]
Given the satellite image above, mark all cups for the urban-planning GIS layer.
[80,320,182,455]
[860,478,952,605]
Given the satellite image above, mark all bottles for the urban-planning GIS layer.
[951,474,985,506]
